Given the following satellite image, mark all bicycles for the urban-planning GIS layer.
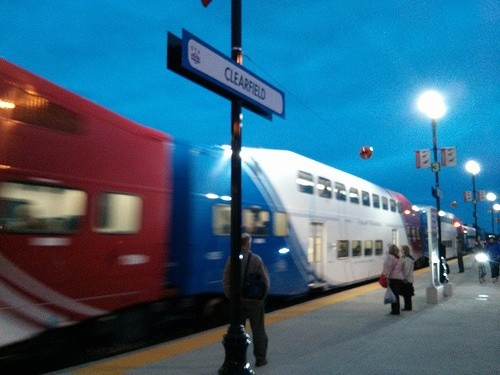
[479,263,487,283]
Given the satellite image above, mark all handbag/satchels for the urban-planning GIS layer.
[384,285,397,304]
[241,278,266,300]
[379,275,388,288]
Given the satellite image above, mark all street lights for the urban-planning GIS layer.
[415,93,457,284]
[485,193,497,234]
[464,161,481,251]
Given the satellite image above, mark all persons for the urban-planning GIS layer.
[455,236,465,273]
[3,193,86,235]
[222,232,270,367]
[475,234,486,283]
[400,244,416,311]
[383,244,404,315]
[483,234,500,283]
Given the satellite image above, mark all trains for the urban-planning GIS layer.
[0,59,496,359]
